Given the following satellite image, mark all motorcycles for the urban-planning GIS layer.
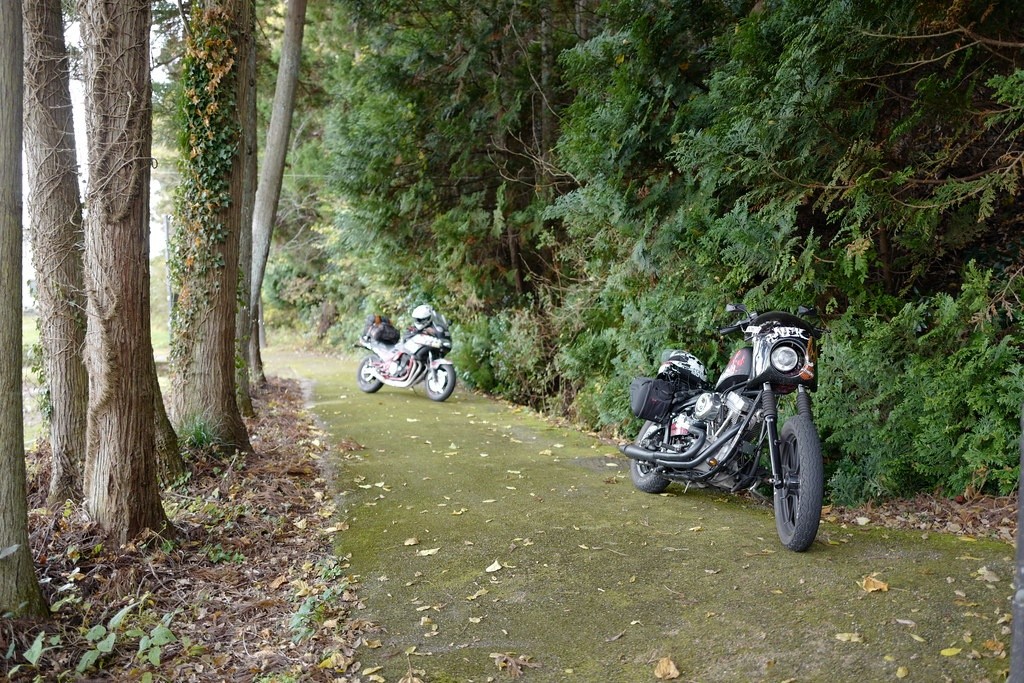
[615,302,826,553]
[351,309,458,402]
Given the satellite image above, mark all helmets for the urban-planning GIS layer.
[657,350,709,386]
[411,303,435,331]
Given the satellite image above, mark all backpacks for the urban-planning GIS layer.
[363,314,400,346]
